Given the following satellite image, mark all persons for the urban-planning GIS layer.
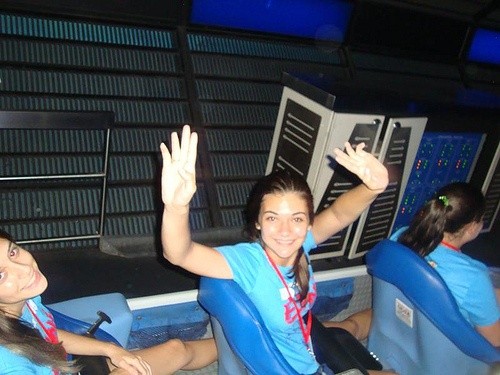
[389,182,500,349]
[160,124,399,375]
[0,228,218,375]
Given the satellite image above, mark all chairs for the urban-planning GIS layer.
[45,307,122,361]
[198,276,300,375]
[364,239,500,375]
